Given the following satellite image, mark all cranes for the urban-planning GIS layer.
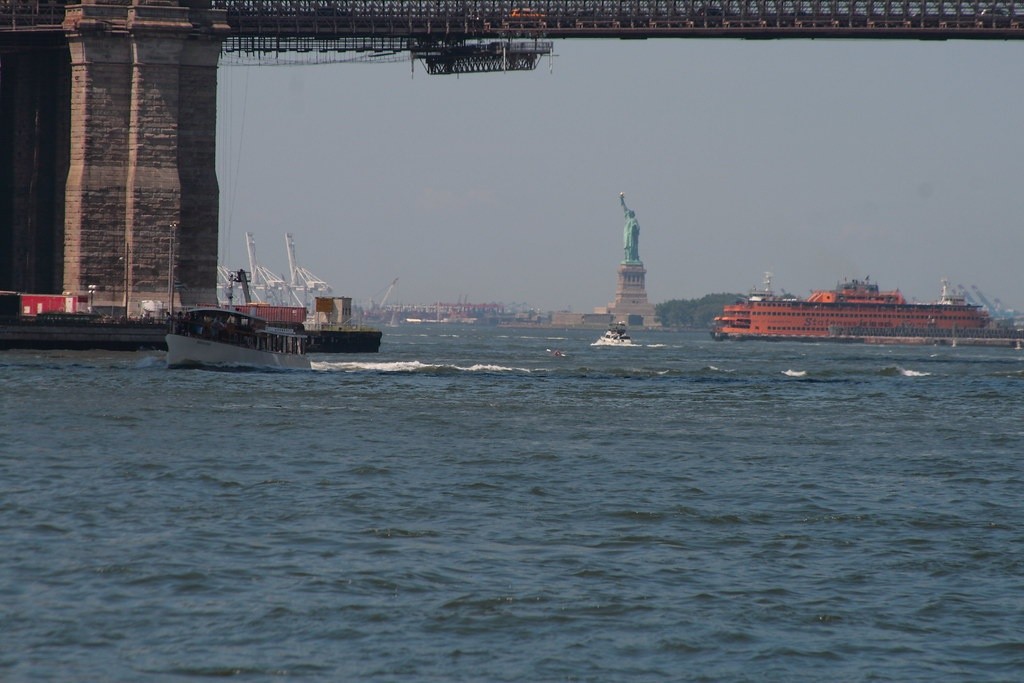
[218,231,333,322]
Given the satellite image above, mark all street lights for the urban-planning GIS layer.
[88,284,98,313]
[168,224,176,315]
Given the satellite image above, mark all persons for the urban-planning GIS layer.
[620,195,640,261]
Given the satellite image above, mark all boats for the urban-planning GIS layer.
[595,320,634,345]
[164,303,316,373]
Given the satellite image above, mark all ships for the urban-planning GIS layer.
[710,270,993,344]
